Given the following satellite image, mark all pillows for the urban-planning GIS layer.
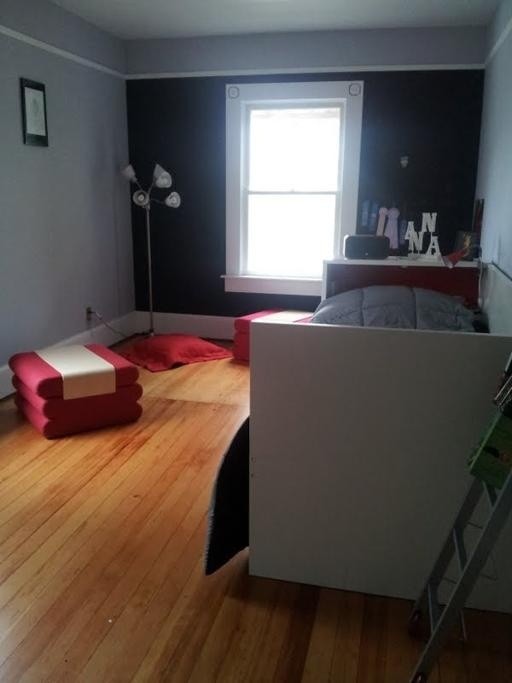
[117,334,234,373]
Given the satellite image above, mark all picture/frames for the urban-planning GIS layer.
[18,76,49,147]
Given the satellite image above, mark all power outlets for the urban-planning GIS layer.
[86,307,92,321]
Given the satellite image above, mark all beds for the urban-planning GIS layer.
[248,256,512,652]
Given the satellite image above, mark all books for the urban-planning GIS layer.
[467,351,511,491]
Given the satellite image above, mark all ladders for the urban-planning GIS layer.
[409,356,512,682]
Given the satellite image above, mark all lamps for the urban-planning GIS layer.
[120,163,182,336]
